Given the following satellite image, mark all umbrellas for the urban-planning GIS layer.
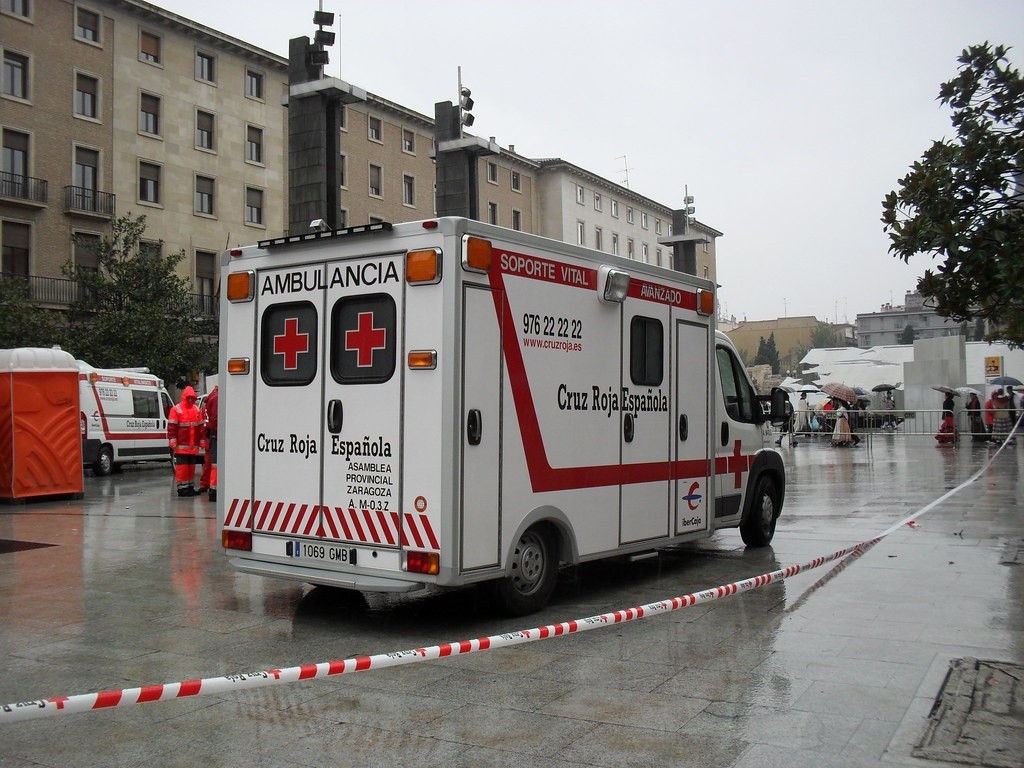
[850,384,870,395]
[797,384,820,402]
[814,398,831,410]
[821,380,857,402]
[774,386,796,393]
[954,387,984,402]
[991,376,1023,385]
[932,385,961,397]
[872,384,895,392]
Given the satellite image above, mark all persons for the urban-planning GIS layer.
[984,386,1021,445]
[775,395,798,445]
[167,385,218,502]
[880,390,897,430]
[934,392,961,445]
[824,396,860,447]
[794,393,811,437]
[965,393,987,442]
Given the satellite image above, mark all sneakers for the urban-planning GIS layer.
[177,482,201,497]
[198,487,207,494]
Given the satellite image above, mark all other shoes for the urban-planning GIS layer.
[775,440,780,444]
[853,438,861,446]
[794,441,798,445]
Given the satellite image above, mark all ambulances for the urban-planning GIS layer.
[215,214,786,615]
[73,367,175,477]
